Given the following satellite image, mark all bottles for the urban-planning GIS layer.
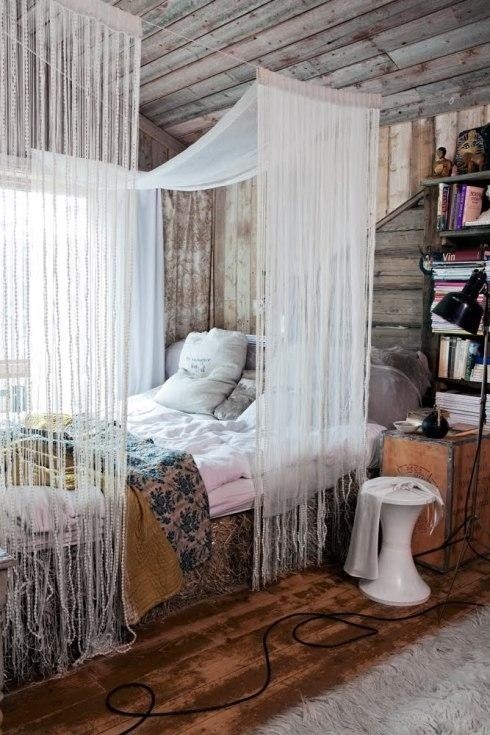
[422,411,449,439]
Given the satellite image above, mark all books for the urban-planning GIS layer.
[430,181,490,428]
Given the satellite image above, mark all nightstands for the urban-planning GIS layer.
[383,422,490,575]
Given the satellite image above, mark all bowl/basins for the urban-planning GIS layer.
[392,421,421,433]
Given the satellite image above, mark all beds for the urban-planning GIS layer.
[0,334,436,698]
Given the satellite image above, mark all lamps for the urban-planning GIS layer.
[412,269,490,628]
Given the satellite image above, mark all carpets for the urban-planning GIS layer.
[242,606,490,735]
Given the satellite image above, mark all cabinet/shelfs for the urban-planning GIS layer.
[423,170,490,435]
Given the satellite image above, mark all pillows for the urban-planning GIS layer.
[157,327,256,420]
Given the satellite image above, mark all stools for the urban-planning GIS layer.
[360,486,433,610]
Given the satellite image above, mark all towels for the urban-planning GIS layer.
[344,474,446,579]
